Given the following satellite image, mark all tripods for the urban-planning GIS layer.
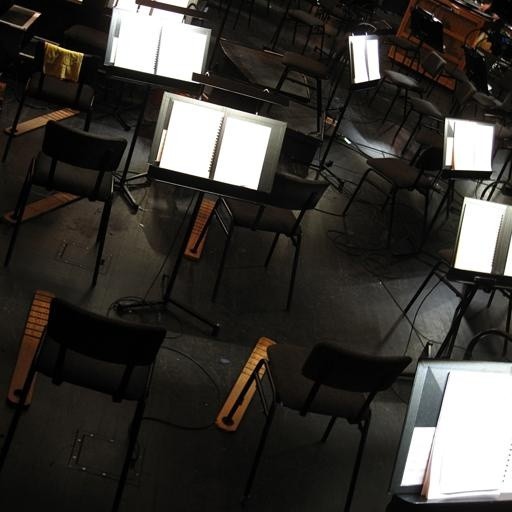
[310,104,347,195]
[115,210,222,340]
[113,93,150,212]
